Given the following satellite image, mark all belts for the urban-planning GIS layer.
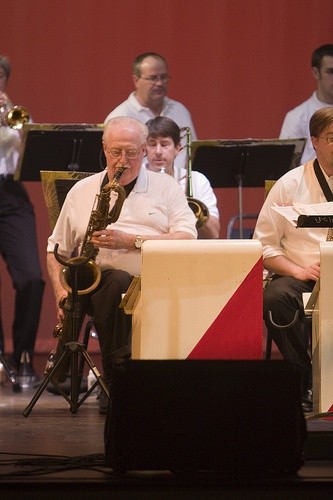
[0,174,14,181]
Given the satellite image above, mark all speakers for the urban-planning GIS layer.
[104,359,307,479]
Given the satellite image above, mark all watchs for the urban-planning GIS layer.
[135,235,143,252]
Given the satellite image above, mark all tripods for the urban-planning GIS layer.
[22,243,111,419]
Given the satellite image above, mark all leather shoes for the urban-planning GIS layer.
[298,368,313,412]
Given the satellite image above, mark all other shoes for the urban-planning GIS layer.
[96,390,110,414]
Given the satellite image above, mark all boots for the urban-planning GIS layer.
[0,309,7,382]
[10,278,46,393]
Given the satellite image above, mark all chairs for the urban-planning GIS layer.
[226,214,296,360]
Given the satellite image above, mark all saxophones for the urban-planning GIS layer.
[178,129,209,231]
[49,167,126,370]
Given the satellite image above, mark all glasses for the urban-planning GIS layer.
[139,74,171,83]
[106,144,142,159]
[317,136,333,145]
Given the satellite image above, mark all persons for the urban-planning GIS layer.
[278,43,333,170]
[47,114,199,415]
[138,116,220,240]
[103,52,197,169]
[252,105,333,413]
[0,56,47,392]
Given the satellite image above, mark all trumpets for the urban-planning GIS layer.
[0,105,31,131]
